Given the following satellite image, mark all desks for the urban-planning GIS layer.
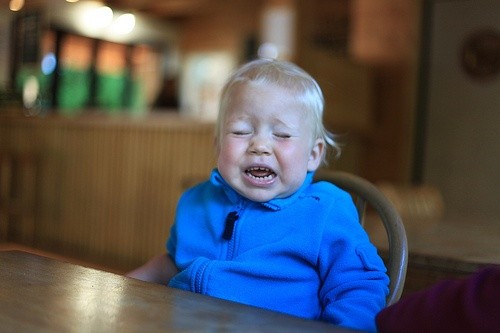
[0,250,359,333]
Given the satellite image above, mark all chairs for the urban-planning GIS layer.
[312,167,408,308]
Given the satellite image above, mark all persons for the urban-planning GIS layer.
[126,58,390,333]
